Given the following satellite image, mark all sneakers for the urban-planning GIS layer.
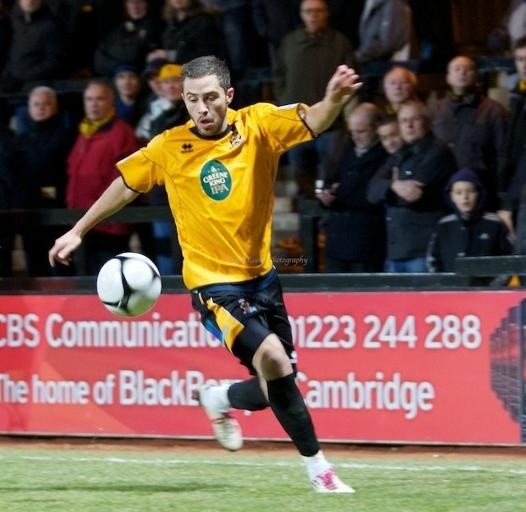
[196,383,243,450]
[312,468,352,492]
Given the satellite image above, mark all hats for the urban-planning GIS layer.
[113,58,182,81]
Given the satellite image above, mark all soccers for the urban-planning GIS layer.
[96,253,162,317]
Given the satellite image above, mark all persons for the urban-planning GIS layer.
[1,1,277,271]
[277,2,526,287]
[49,55,365,494]
